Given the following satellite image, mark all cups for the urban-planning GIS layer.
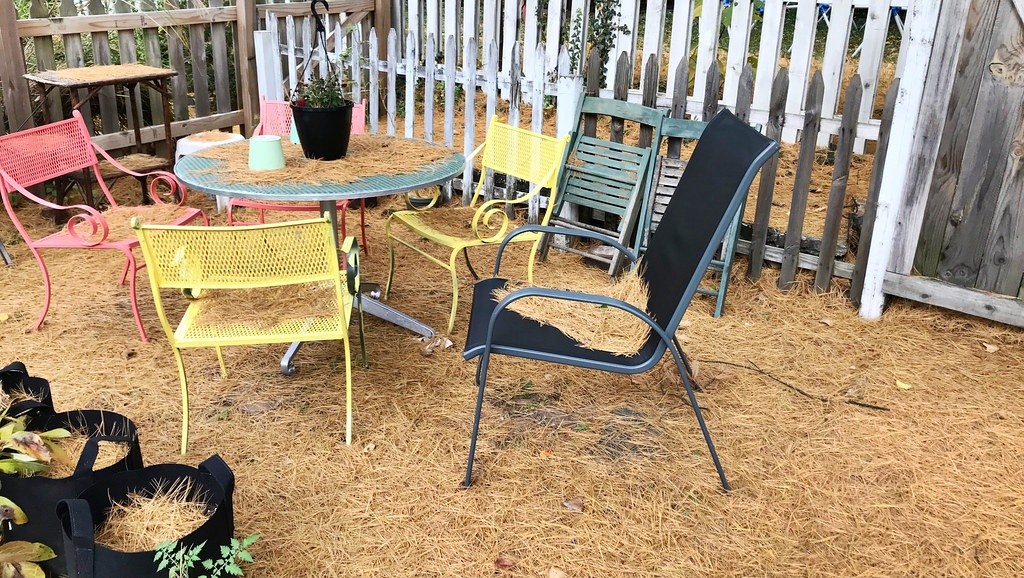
[248,135,286,173]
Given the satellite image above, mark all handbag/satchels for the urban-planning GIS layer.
[0,362,236,578]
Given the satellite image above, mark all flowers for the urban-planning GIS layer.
[287,73,354,111]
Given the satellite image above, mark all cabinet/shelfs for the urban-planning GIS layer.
[22,64,181,227]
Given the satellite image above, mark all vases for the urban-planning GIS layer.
[292,99,352,161]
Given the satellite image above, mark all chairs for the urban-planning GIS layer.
[0,120,210,344]
[130,207,366,456]
[228,96,367,274]
[461,109,778,488]
[644,112,765,317]
[537,91,672,278]
[382,117,572,334]
